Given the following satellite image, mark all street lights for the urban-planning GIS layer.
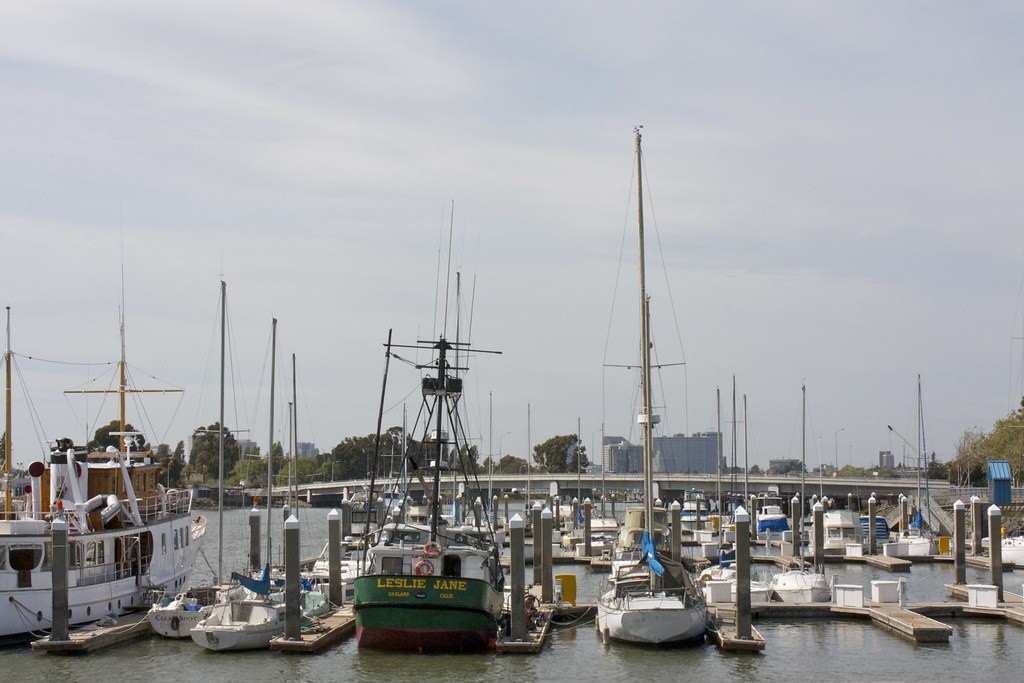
[887,423,921,532]
[500,432,511,474]
[835,428,846,479]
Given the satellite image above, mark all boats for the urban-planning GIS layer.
[354,193,506,652]
[0,270,210,642]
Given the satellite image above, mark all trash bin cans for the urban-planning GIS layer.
[939,536,950,555]
[554,574,577,608]
[1001,528,1007,539]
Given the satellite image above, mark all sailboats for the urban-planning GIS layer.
[150,279,330,651]
[594,123,712,646]
[772,385,831,603]
[896,371,938,558]
[699,374,776,608]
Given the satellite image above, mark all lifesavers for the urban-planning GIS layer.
[170,617,180,630]
[701,574,712,582]
[423,540,442,558]
[413,560,434,576]
